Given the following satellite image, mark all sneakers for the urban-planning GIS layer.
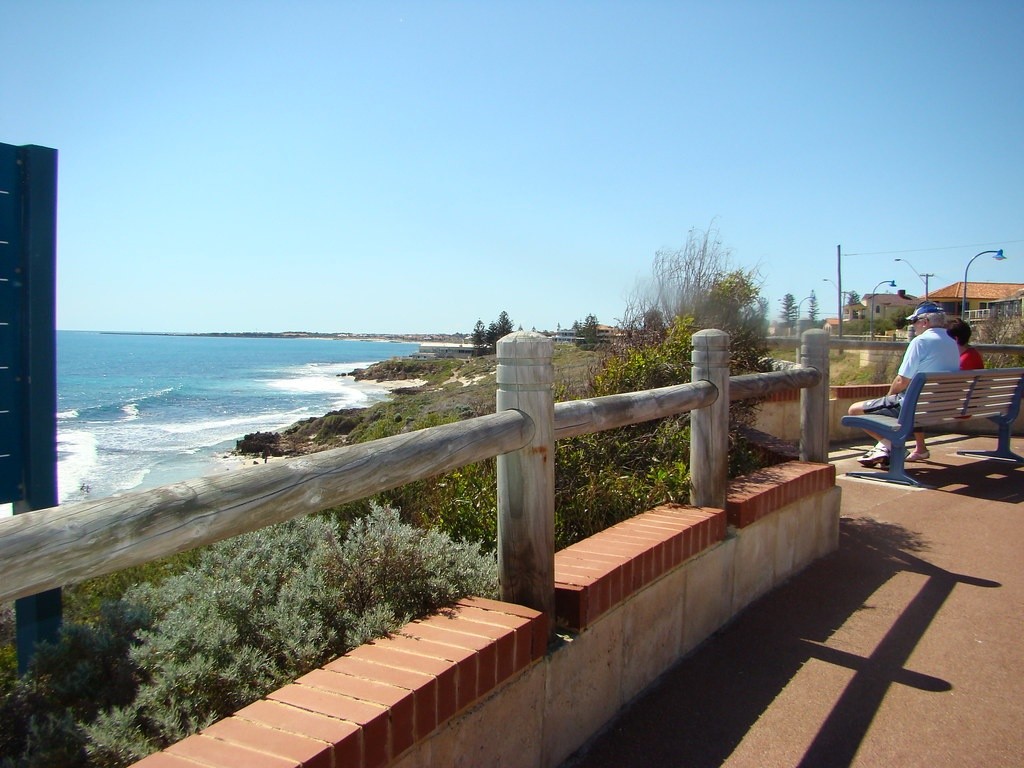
[880,447,910,469]
[857,447,889,468]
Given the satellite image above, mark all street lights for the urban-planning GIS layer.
[894,258,929,301]
[822,278,846,321]
[870,280,897,337]
[960,248,1007,323]
[798,296,816,320]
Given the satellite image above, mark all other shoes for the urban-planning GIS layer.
[904,450,930,462]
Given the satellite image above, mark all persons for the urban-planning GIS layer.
[904,317,984,462]
[848,300,960,468]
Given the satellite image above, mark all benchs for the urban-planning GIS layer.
[840,365,1024,487]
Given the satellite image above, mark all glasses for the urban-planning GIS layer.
[909,318,929,325]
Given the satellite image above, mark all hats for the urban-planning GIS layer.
[906,301,943,320]
